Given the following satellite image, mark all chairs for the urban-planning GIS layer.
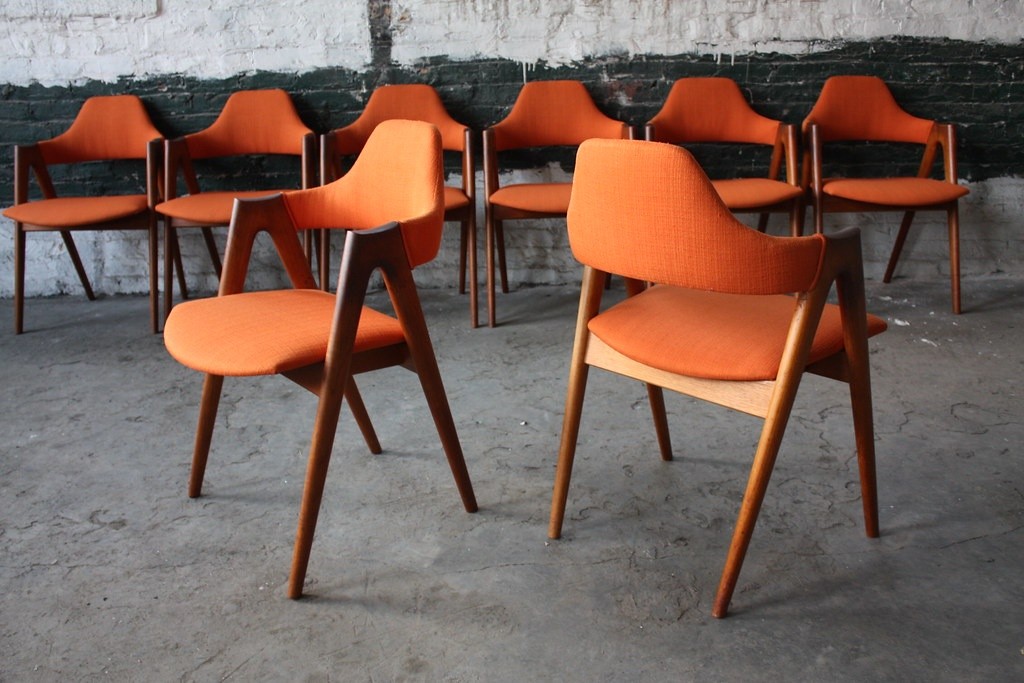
[549,140,893,621]
[645,79,804,240]
[162,89,314,317]
[0,93,161,329]
[480,81,634,327]
[162,117,479,598]
[317,83,481,328]
[801,76,968,314]
